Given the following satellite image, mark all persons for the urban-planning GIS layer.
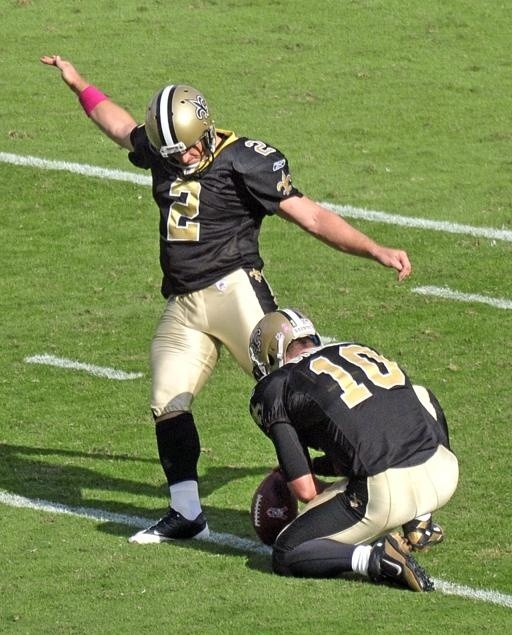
[246,304,462,596]
[38,46,412,545]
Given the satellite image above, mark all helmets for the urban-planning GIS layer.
[248,308,323,381]
[145,83,216,157]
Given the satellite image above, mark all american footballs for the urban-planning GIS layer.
[250,472,296,543]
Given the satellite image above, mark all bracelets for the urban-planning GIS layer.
[76,82,109,118]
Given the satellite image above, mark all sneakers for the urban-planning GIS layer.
[366,530,435,593]
[402,513,444,551]
[127,506,212,543]
